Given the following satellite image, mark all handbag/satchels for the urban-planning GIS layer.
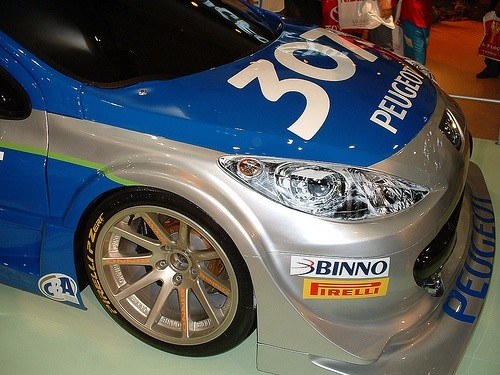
[338,0,395,30]
[476,11,500,61]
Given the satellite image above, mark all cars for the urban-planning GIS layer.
[0,0,497,375]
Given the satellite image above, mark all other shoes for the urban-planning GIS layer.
[477,67,500,78]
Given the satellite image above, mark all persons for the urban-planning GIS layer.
[397,0,432,67]
[475,0,500,79]
[336,0,394,53]
